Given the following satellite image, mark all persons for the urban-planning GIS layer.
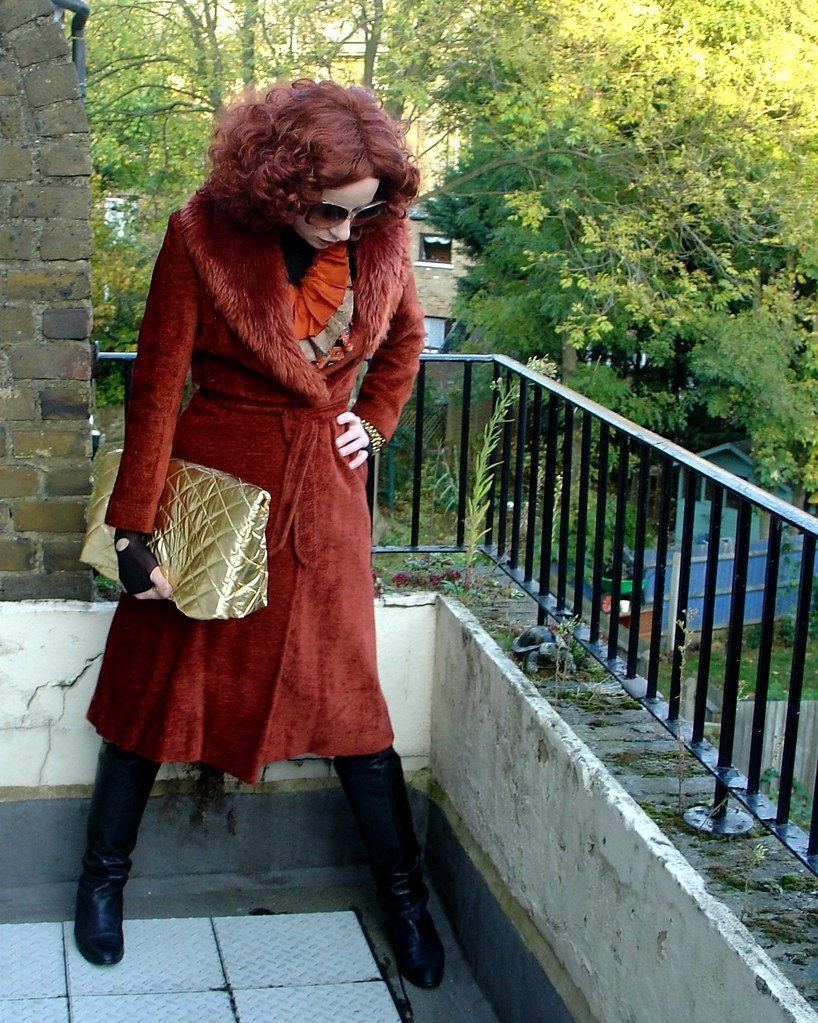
[73,81,447,989]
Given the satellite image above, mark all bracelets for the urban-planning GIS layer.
[361,419,382,456]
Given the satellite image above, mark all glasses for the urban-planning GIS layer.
[286,200,386,229]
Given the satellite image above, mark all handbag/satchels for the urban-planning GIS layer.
[79,449,271,621]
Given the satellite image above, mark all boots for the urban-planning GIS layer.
[332,747,445,989]
[74,737,162,965]
